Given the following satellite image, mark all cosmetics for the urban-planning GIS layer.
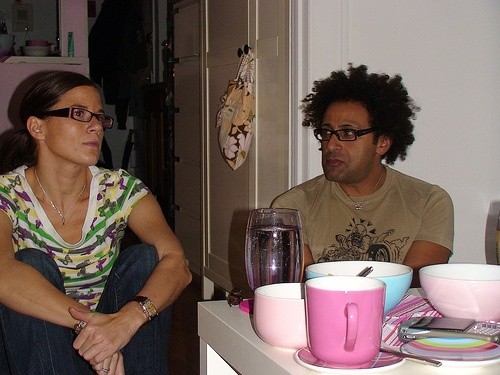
[68,32,74,57]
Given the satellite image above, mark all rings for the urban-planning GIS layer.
[102,368,108,373]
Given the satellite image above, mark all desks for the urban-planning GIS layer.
[197,288,500,375]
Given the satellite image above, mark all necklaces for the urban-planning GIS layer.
[341,170,384,210]
[34,168,87,225]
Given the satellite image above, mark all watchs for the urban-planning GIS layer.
[134,295,158,321]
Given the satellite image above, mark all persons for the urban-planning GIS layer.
[0,71,192,375]
[272,64,456,290]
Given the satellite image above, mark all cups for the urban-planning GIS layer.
[304,276,386,365]
[244,207,305,292]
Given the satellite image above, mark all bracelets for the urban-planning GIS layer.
[75,321,87,334]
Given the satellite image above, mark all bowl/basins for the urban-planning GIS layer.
[305,260,413,314]
[419,263,500,322]
[20,45,50,57]
[0,34,15,55]
[254,282,307,350]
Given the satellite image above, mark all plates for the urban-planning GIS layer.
[294,347,406,373]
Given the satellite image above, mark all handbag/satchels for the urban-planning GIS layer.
[217,49,255,171]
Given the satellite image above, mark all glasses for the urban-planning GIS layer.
[313,126,379,141]
[37,107,113,129]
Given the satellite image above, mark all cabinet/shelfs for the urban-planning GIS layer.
[173,0,201,280]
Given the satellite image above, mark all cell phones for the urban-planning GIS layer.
[399,316,500,345]
